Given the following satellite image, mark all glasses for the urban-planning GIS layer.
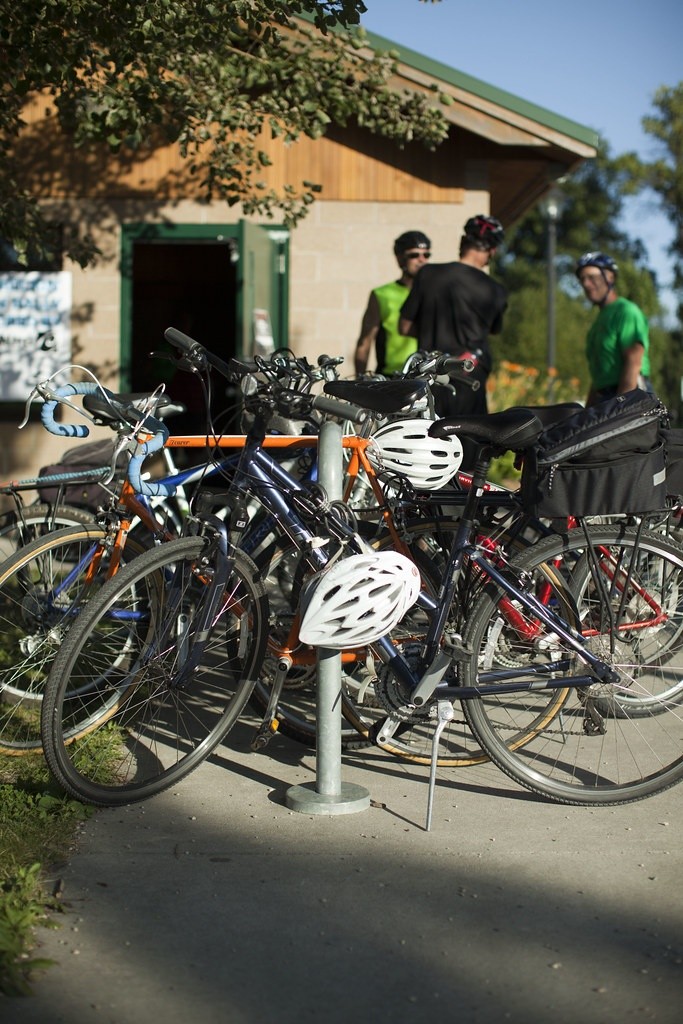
[408,253,430,258]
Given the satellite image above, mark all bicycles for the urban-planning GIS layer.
[1,326,683,831]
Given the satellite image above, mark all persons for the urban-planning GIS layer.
[574,249,654,409]
[398,214,508,475]
[353,230,432,381]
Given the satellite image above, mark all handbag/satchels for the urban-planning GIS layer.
[514,389,671,517]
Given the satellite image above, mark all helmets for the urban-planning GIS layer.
[575,251,618,288]
[297,551,421,650]
[365,418,464,491]
[464,215,504,249]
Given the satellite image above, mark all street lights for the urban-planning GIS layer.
[537,187,567,406]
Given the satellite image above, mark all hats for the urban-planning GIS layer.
[394,231,430,255]
